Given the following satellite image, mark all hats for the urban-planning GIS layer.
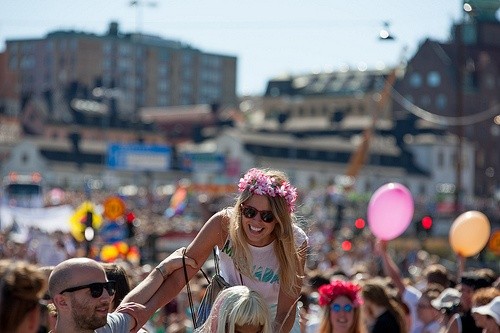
[471,295,500,326]
[430,288,461,309]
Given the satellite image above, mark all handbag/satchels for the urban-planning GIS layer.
[181,244,233,328]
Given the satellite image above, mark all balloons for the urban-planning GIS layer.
[367,182,414,240]
[449,210,491,258]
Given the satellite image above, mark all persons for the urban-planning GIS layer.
[362,283,405,333]
[97,262,149,333]
[471,286,500,333]
[0,184,500,305]
[116,168,309,333]
[374,236,449,333]
[0,258,48,333]
[48,247,197,332]
[298,291,311,333]
[429,288,462,333]
[317,280,369,333]
[411,288,443,333]
[304,273,332,333]
[194,285,273,333]
[473,295,500,333]
[457,272,491,333]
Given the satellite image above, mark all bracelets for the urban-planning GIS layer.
[155,264,169,281]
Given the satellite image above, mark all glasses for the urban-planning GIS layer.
[331,304,354,312]
[60,280,115,298]
[241,203,275,223]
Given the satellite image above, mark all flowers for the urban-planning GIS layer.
[238,169,299,212]
[317,281,365,308]
